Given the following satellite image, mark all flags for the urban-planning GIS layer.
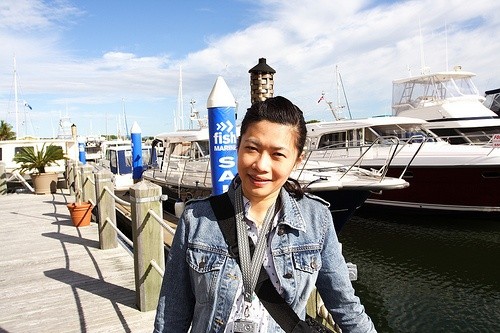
[24,102,33,110]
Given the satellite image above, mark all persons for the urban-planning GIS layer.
[153,95,380,333]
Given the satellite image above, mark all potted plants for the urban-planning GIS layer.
[12,142,70,194]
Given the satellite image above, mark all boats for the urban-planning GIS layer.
[138,133,426,239]
[94,140,161,203]
[313,62,500,129]
[282,115,500,220]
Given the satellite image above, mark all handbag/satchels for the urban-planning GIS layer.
[293,313,334,333]
